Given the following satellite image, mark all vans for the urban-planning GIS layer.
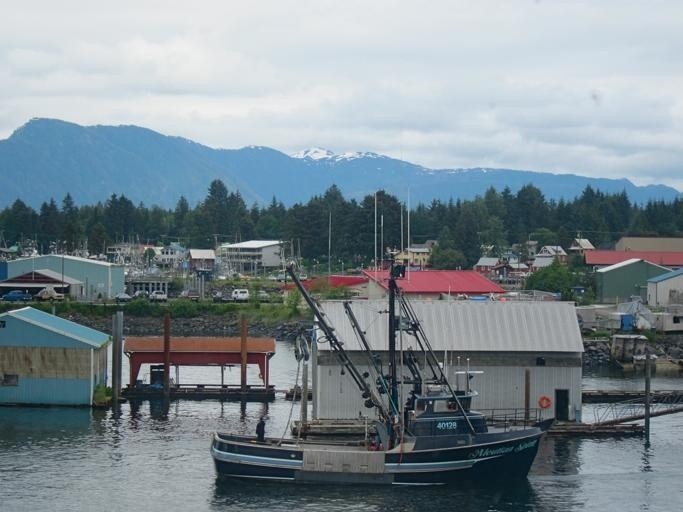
[251,288,270,302]
[231,288,249,304]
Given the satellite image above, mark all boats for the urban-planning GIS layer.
[208,257,554,487]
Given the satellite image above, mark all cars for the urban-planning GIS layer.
[114,289,201,303]
[1,290,32,302]
[34,289,63,302]
